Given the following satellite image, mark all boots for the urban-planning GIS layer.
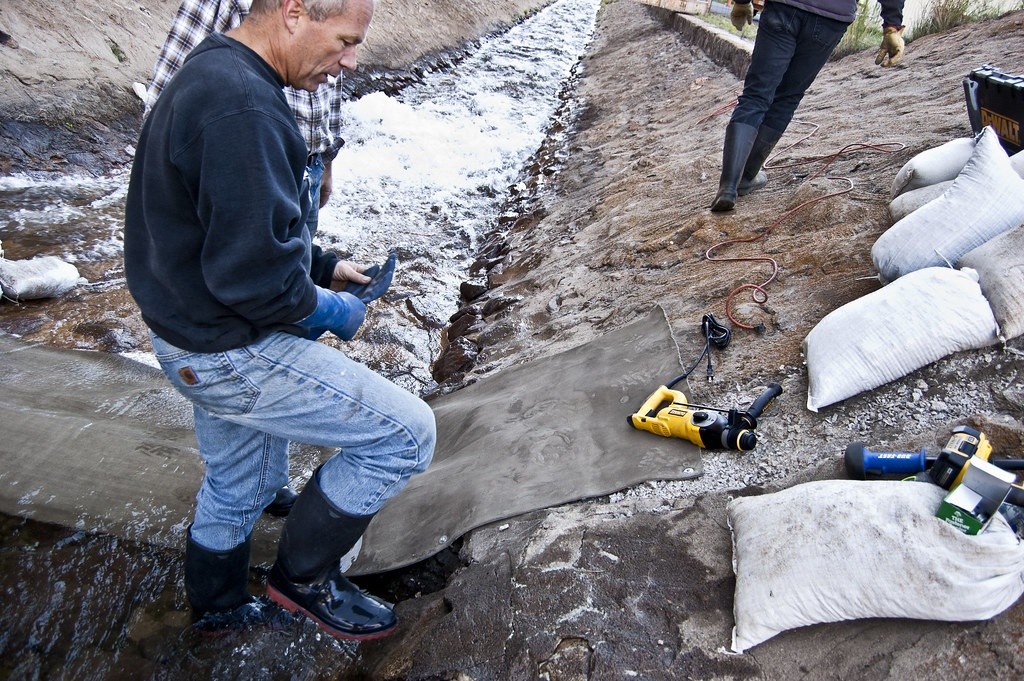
[265,464,398,640]
[735,125,782,195]
[711,123,758,212]
[183,524,270,640]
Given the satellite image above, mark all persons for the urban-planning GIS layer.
[122,0,438,642]
[707,0,908,213]
[140,0,343,243]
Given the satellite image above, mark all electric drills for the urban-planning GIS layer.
[926,423,1023,508]
[625,380,784,453]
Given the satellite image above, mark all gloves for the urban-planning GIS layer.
[307,254,398,340]
[290,282,365,341]
[875,24,905,67]
[730,3,753,31]
